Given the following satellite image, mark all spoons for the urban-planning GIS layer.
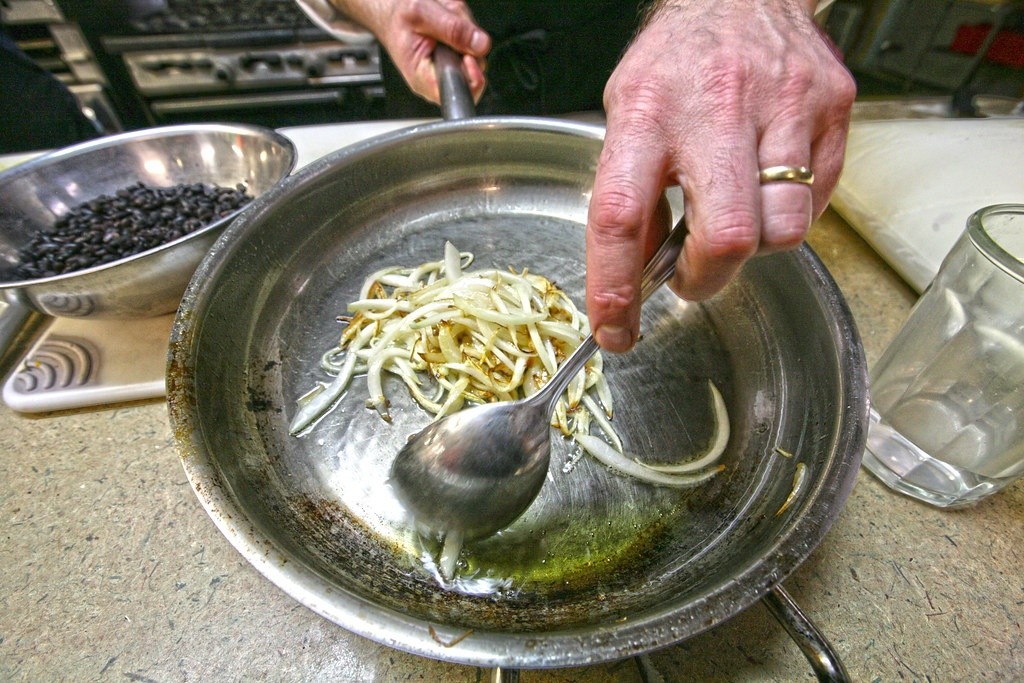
[392,214,691,542]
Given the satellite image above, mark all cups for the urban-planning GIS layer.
[861,204,1024,512]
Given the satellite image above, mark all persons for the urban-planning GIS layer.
[296,0,856,356]
[0,1,106,153]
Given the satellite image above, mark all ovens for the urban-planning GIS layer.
[150,86,386,130]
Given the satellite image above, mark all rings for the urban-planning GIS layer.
[759,165,813,186]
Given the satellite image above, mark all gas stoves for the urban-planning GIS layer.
[92,0,388,99]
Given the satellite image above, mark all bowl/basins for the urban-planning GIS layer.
[0,121,297,321]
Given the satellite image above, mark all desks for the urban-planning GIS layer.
[0,93,1024,683]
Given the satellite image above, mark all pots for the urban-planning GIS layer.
[164,42,872,670]
